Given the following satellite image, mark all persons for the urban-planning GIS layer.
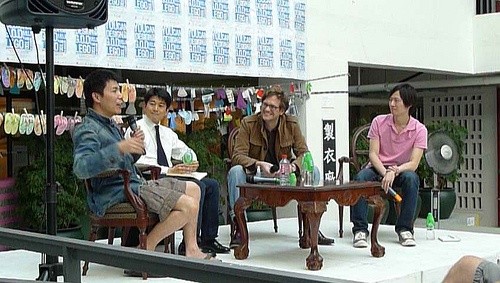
[73,69,225,277]
[442,255,500,283]
[350,84,428,248]
[226,89,334,248]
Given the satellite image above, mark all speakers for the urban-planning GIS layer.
[0,0,109,34]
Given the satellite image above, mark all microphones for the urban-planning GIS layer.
[376,176,402,202]
[271,165,278,174]
[246,175,280,185]
[127,116,146,155]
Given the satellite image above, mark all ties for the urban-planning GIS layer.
[155,126,170,167]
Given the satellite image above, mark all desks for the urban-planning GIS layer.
[234,180,387,270]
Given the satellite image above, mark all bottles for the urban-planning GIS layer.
[182,150,193,175]
[426,213,436,241]
[302,151,314,188]
[279,154,292,186]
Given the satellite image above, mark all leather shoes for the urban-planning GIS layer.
[178,236,216,257]
[200,239,230,253]
[229,230,240,248]
[318,230,334,244]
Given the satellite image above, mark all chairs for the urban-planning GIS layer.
[228,126,303,237]
[82,164,168,283]
[337,122,401,239]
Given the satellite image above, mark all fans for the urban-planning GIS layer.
[423,130,459,230]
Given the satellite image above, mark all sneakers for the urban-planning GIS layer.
[399,230,416,246]
[353,230,368,247]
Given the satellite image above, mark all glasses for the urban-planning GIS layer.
[261,102,279,110]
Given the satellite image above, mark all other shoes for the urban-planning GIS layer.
[202,255,222,262]
[124,270,141,277]
